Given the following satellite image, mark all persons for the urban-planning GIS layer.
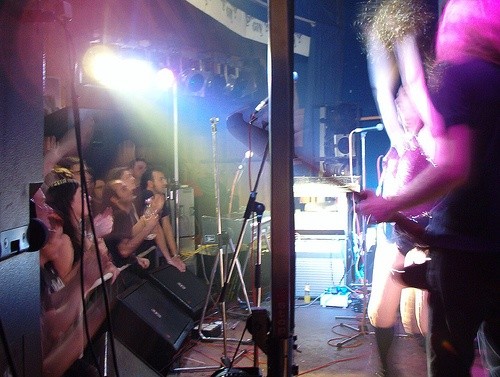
[352,0,500,377]
[30,117,186,351]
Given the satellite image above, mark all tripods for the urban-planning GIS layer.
[170,118,412,377]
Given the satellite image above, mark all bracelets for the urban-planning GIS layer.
[174,253,178,257]
[88,233,94,242]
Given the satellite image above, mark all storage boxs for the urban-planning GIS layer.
[192,323,221,342]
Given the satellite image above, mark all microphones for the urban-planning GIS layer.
[249,71,298,119]
[354,123,383,132]
[9,9,55,21]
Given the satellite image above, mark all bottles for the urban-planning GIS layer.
[304,283,311,303]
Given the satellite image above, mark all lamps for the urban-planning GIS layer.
[121,50,261,97]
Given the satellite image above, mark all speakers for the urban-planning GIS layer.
[110,279,195,373]
[150,264,214,319]
[321,104,362,176]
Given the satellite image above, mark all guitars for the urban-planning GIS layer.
[224,111,437,293]
